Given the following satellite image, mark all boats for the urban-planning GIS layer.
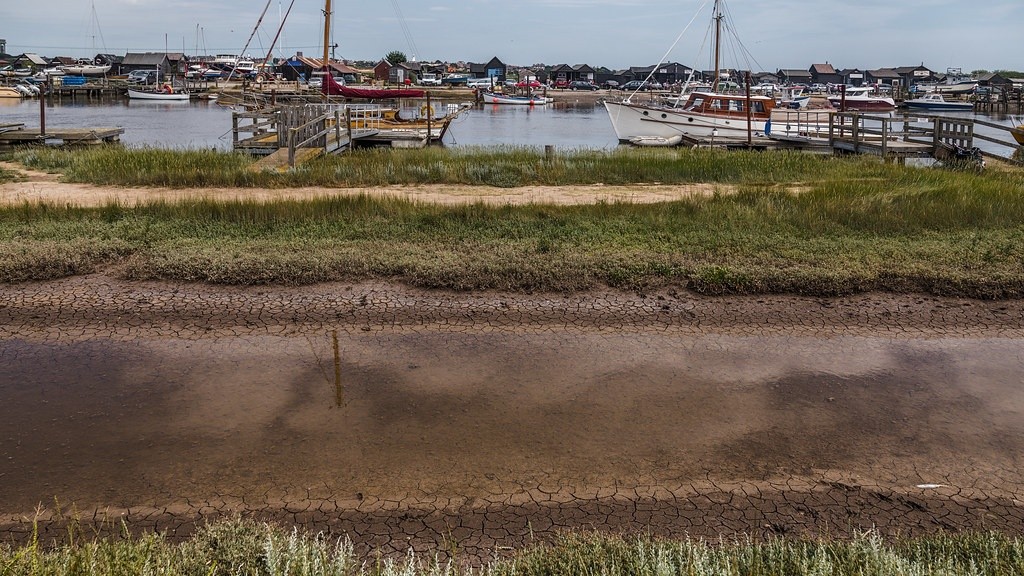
[0,0,1024,148]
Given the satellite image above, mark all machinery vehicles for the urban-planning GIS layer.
[255,64,284,84]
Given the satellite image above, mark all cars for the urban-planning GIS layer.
[810,82,827,92]
[605,79,619,90]
[877,83,893,95]
[751,82,779,95]
[307,77,323,89]
[467,78,497,90]
[504,79,517,87]
[343,74,356,84]
[831,83,846,92]
[514,79,542,88]
[569,80,600,92]
[788,82,812,93]
[333,76,346,86]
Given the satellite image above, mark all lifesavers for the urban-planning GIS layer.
[422,104,435,117]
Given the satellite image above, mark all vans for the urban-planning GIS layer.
[619,80,650,92]
[131,69,165,86]
